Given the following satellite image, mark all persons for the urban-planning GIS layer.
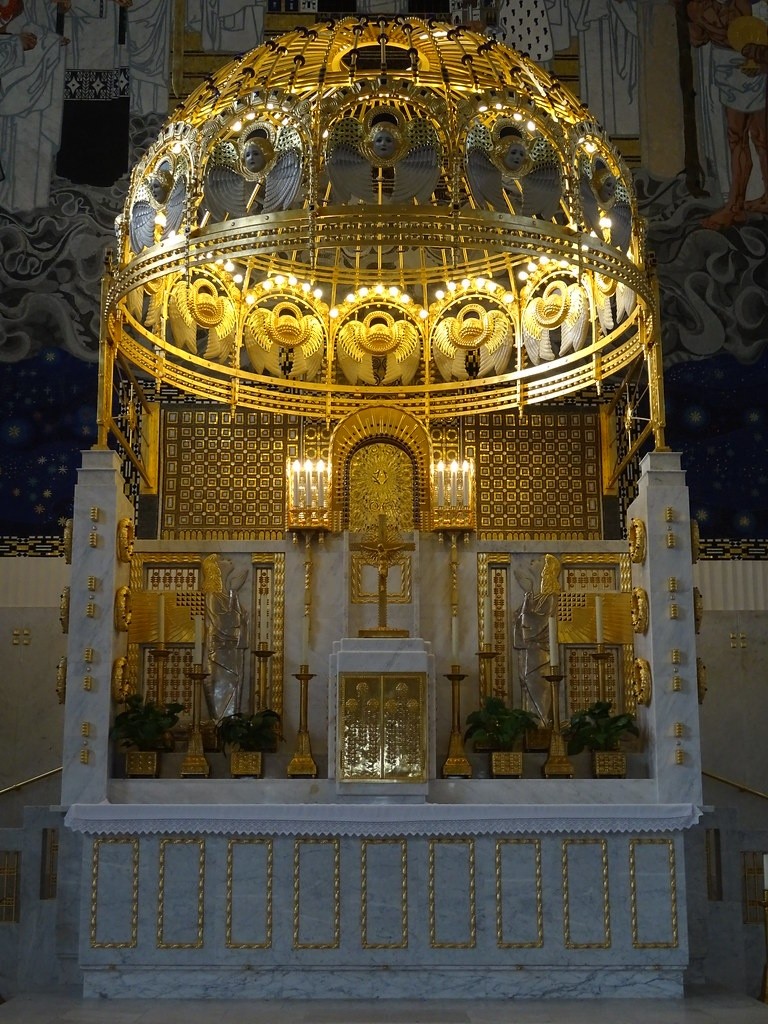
[513,554,562,728]
[203,553,251,727]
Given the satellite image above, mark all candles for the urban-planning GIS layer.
[437,460,445,506]
[462,459,470,506]
[300,615,309,665]
[548,615,560,666]
[595,595,604,643]
[451,615,459,666]
[158,593,165,642]
[315,459,326,507]
[303,460,314,508]
[450,459,457,506]
[260,595,267,642]
[194,615,203,664]
[291,460,301,507]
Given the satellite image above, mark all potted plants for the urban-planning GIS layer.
[562,700,640,779]
[109,693,187,778]
[464,694,541,778]
[213,710,283,780]
